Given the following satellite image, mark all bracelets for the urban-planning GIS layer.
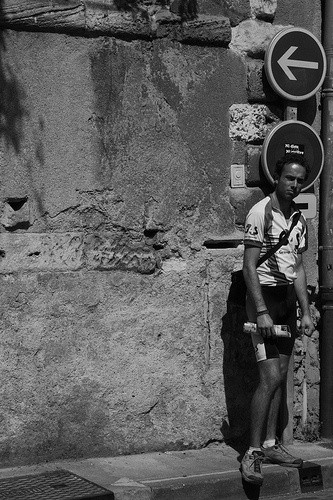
[255,309,270,317]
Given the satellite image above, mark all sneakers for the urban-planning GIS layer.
[260,444,303,468]
[240,450,264,485]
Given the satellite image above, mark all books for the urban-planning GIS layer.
[242,322,291,338]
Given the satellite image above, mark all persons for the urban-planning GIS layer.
[239,152,316,486]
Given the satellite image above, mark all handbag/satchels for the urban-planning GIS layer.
[229,271,250,308]
